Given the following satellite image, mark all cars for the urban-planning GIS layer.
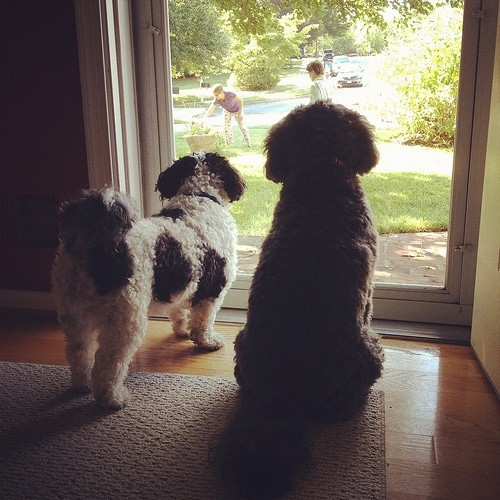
[324,48,365,87]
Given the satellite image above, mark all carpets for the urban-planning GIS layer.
[0,361,387,500]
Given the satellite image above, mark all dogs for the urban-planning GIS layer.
[232,98,386,423]
[49,150,247,410]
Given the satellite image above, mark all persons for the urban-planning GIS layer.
[199,83,252,148]
[306,60,333,105]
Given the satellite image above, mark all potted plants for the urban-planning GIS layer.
[184,121,215,153]
